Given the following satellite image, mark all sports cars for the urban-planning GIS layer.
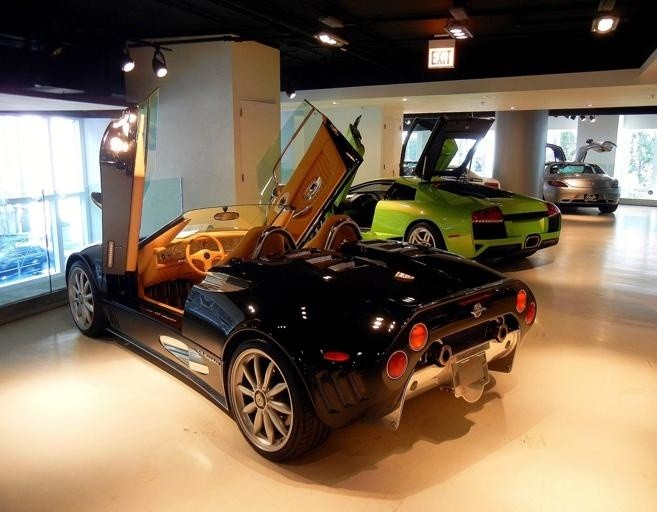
[540,138,624,213]
[396,116,501,189]
[322,111,565,262]
[65,92,543,468]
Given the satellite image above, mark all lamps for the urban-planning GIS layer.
[443,20,474,40]
[590,10,621,34]
[151,49,168,78]
[311,31,349,52]
[286,89,297,99]
[119,54,136,73]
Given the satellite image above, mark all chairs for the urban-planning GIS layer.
[215,224,285,263]
[301,214,363,251]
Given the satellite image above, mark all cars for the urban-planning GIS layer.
[0,232,55,283]
